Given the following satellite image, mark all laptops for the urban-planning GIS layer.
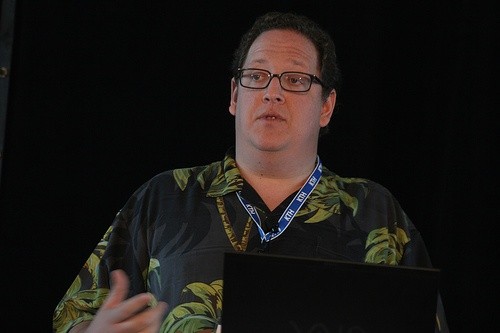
[222,251,440,333]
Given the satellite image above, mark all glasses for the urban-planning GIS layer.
[237,67,324,93]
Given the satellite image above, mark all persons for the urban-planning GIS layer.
[52,10,450,333]
